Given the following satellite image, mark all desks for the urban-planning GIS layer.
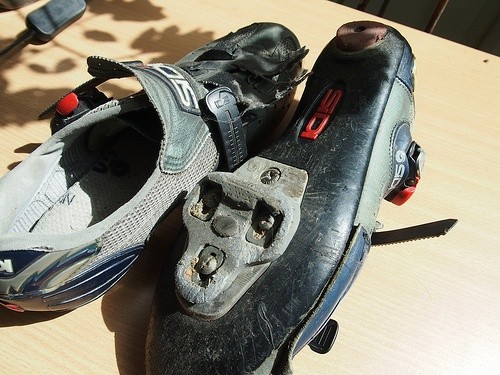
[1,0,500,370]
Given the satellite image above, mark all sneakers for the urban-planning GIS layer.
[0,23,306,314]
[143,21,423,374]
